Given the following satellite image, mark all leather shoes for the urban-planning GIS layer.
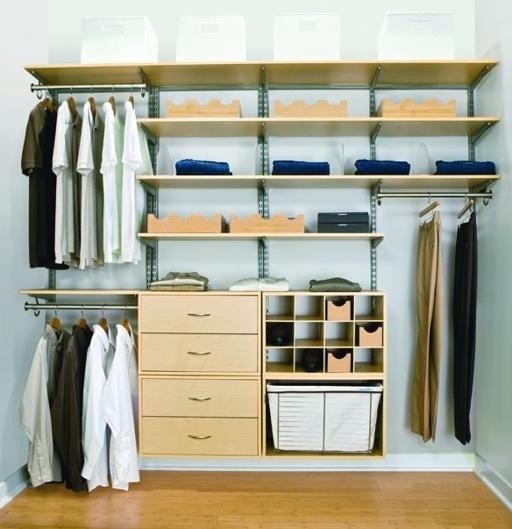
[270,323,289,345]
[303,349,322,371]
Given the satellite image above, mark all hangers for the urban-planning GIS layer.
[417,196,479,220]
[41,81,135,119]
[49,304,131,334]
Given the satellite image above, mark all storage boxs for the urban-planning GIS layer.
[326,300,351,321]
[356,327,382,347]
[327,351,351,373]
[267,380,383,452]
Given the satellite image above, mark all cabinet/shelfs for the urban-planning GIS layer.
[261,290,388,472]
[138,291,261,472]
[24,58,503,249]
